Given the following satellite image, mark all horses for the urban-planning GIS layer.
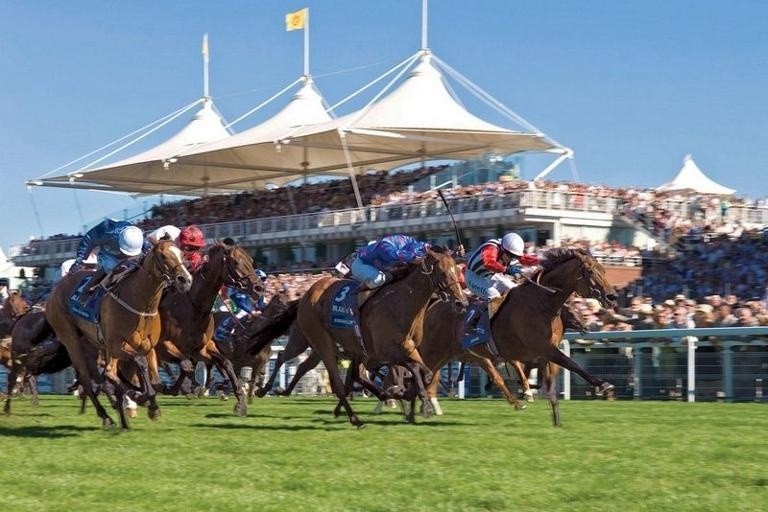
[0,237,619,430]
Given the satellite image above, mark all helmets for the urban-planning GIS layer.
[178,225,206,247]
[118,226,144,257]
[502,233,525,257]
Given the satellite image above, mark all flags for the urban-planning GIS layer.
[286,9,306,31]
